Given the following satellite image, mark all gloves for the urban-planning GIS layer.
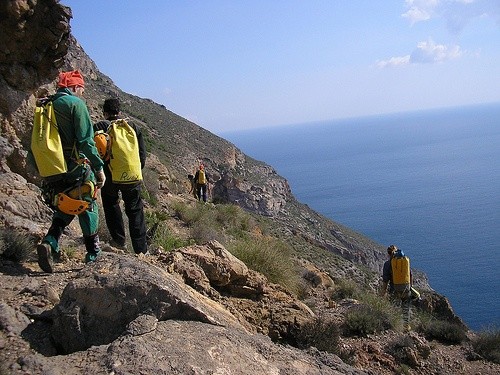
[96,169,106,188]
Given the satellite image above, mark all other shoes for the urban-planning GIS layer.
[110,238,126,250]
[37,243,53,274]
[403,325,411,333]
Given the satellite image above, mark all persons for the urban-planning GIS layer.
[29,70,106,273]
[382,245,412,332]
[188,165,209,202]
[94,98,148,255]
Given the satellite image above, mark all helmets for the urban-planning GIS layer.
[95,134,107,158]
[56,194,87,215]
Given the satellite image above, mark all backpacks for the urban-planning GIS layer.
[199,170,206,184]
[104,119,144,186]
[31,92,67,178]
[391,255,411,297]
